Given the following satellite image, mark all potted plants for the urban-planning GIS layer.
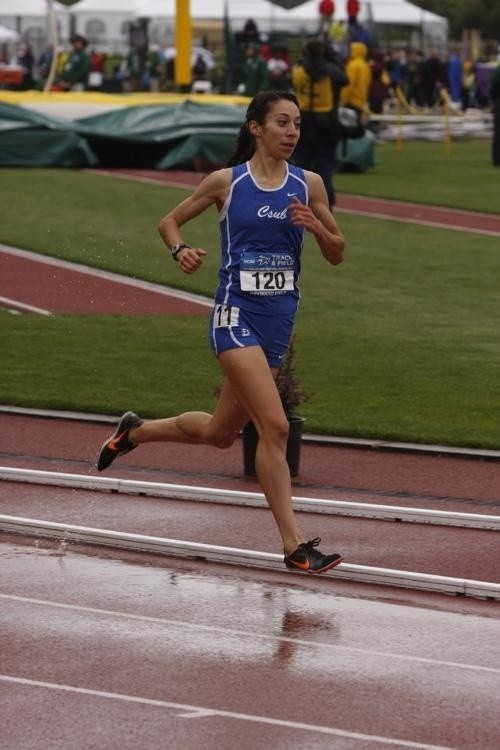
[242,334,309,477]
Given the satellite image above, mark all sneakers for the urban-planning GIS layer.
[98,411,143,471]
[284,537,343,574]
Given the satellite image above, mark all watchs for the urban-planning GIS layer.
[171,243,190,261]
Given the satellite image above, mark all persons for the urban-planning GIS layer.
[7,33,294,98]
[96,91,345,575]
[343,41,499,115]
[282,41,349,211]
